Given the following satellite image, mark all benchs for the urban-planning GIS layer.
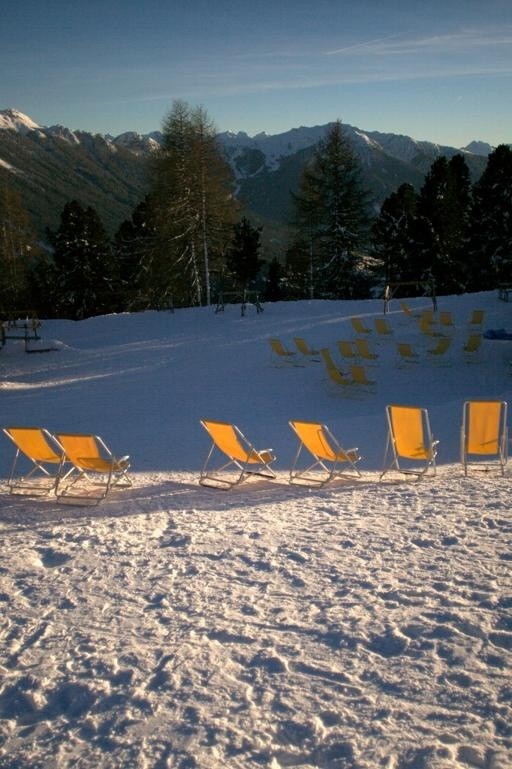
[498,283,512,303]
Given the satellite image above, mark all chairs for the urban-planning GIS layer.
[52,431,134,503]
[376,403,441,484]
[459,399,509,475]
[195,419,277,491]
[3,427,91,493]
[268,302,487,393]
[287,419,362,487]
